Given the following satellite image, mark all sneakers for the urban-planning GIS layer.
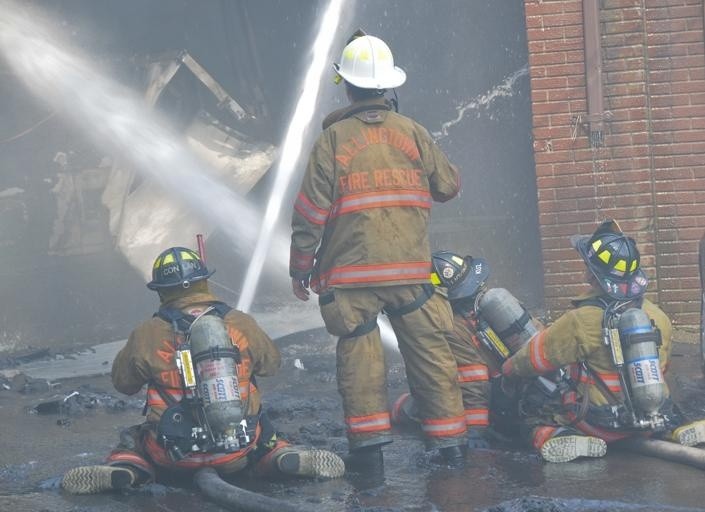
[541,427,608,463]
[61,464,136,495]
[439,445,466,467]
[662,420,705,448]
[276,444,383,479]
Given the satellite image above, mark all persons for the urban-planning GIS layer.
[501,218,704,463]
[388,250,547,450]
[289,27,471,480]
[61,247,346,494]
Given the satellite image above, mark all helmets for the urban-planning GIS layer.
[431,249,490,301]
[575,218,648,301]
[146,247,215,290]
[332,35,407,89]
